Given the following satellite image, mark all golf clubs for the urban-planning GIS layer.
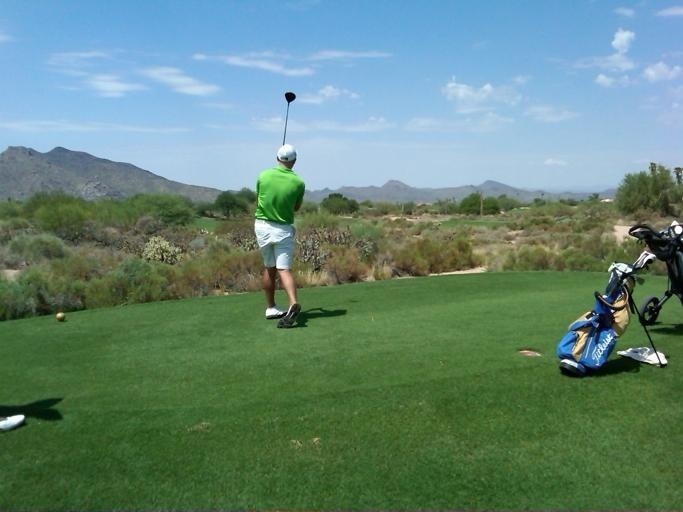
[282,92,296,146]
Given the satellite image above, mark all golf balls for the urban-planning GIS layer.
[57,313,65,322]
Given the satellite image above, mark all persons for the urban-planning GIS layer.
[254,143,306,329]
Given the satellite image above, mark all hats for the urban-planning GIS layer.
[277,143,297,163]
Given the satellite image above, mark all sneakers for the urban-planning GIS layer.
[276,303,301,329]
[265,305,288,319]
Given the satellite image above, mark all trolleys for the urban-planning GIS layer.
[625,222,682,325]
